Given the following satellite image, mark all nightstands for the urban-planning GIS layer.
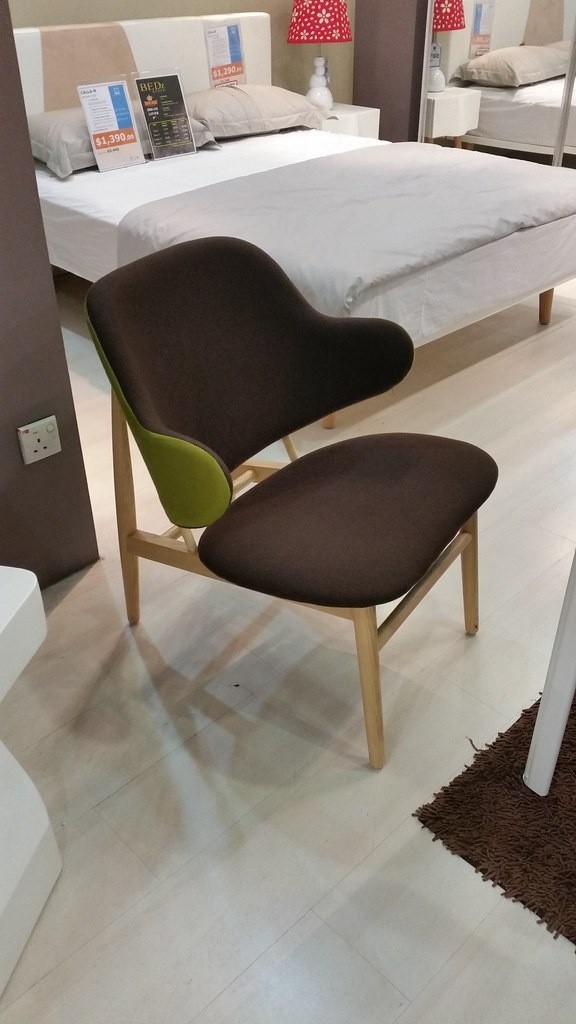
[424,87,481,148]
[324,102,380,138]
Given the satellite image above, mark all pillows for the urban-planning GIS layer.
[184,83,324,140]
[546,40,571,51]
[28,99,222,179]
[449,45,570,87]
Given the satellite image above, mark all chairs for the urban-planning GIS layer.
[83,236,499,769]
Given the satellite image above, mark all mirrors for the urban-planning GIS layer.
[418,0,576,169]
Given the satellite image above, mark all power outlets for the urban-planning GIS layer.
[17,414,61,465]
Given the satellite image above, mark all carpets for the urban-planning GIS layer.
[410,688,576,946]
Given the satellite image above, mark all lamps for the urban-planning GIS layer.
[287,0,352,111]
[428,0,465,92]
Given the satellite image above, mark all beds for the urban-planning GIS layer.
[13,13,576,430]
[442,0,576,167]
